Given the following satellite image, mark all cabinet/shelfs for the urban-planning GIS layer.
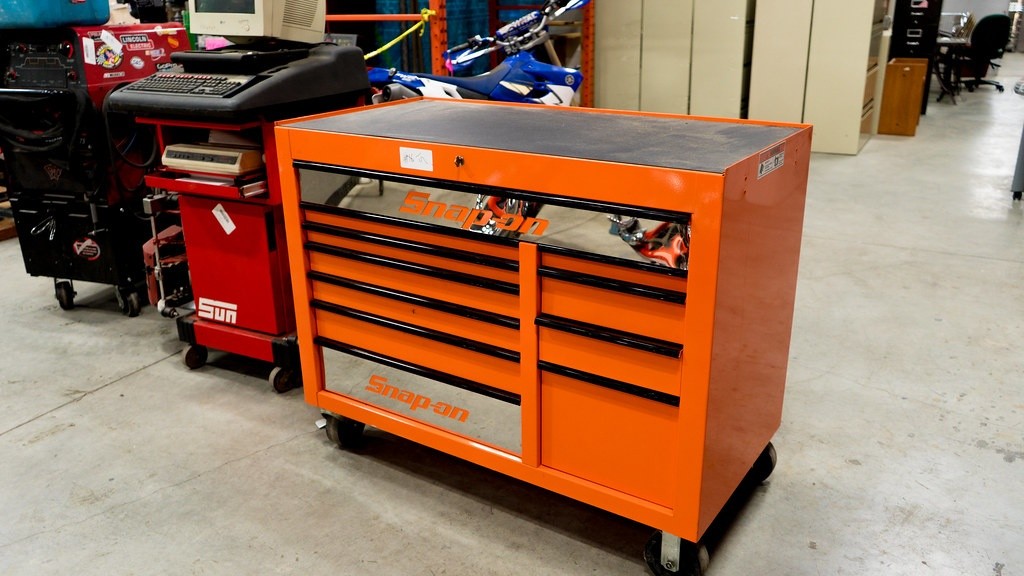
[594,0,887,151]
[877,57,928,136]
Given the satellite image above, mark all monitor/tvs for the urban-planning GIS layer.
[188,0,326,52]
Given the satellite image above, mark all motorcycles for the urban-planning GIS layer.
[366,0,589,110]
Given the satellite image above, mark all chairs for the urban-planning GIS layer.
[948,14,1011,92]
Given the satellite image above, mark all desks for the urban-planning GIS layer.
[935,37,968,98]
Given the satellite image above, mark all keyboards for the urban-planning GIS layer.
[121,73,256,98]
